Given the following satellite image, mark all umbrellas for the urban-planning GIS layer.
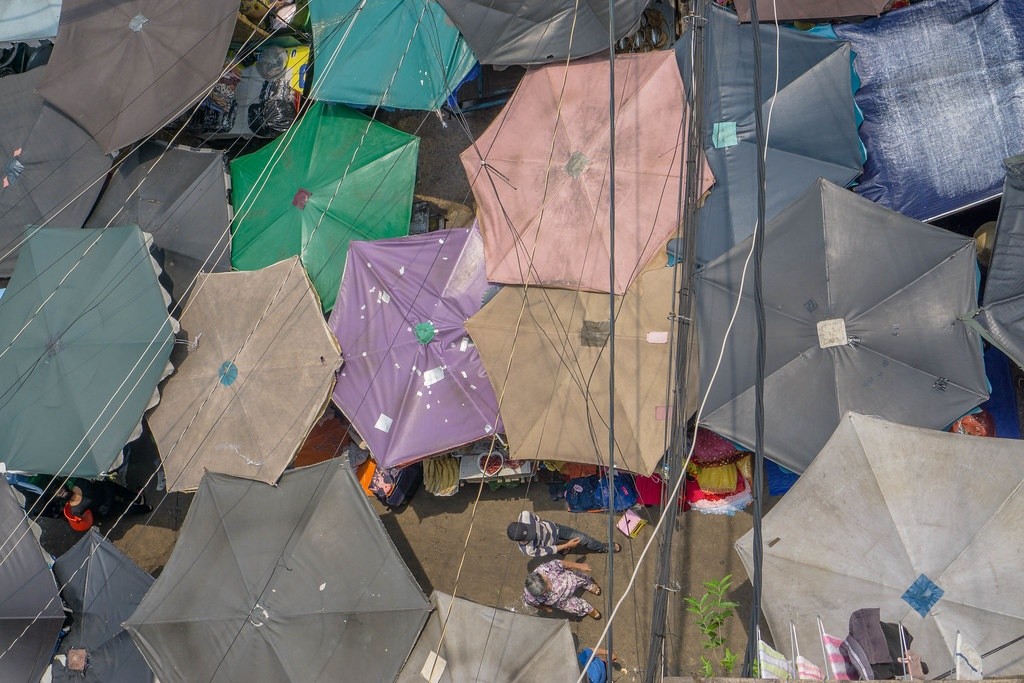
[0,0,1024,683]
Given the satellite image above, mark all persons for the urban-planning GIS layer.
[577,647,621,683]
[51,481,153,519]
[521,559,602,620]
[239,0,284,21]
[507,511,621,559]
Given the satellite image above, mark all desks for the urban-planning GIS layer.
[460,453,532,485]
[193,44,309,139]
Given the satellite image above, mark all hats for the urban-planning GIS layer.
[507,522,535,541]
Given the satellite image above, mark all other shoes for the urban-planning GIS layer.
[148,504,153,511]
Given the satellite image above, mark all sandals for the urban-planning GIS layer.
[599,543,621,553]
[588,608,602,620]
[587,582,601,595]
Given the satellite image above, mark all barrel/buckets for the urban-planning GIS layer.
[63,502,93,531]
